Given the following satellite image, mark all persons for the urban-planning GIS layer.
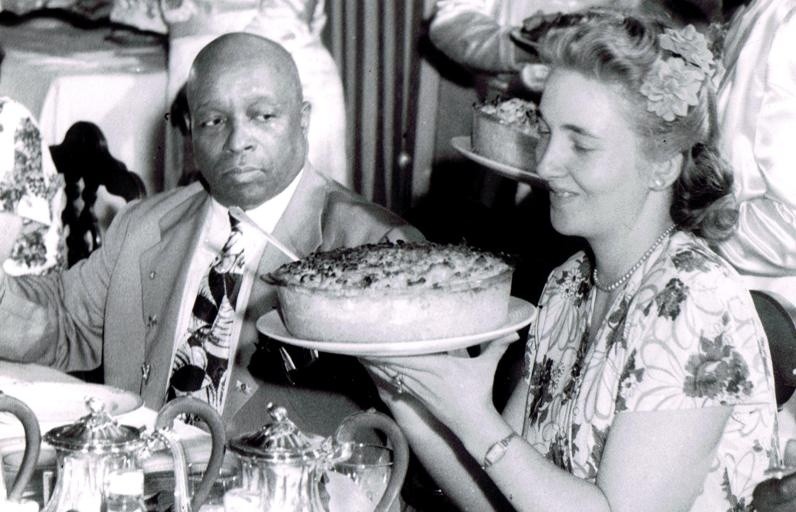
[428,1,668,303]
[157,0,349,191]
[693,0,796,512]
[0,96,68,278]
[353,5,780,512]
[0,32,425,436]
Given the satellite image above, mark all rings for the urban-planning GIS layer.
[393,372,406,395]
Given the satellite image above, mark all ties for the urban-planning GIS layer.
[167,211,245,416]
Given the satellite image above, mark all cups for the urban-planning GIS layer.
[327,442,402,511]
[2,449,59,503]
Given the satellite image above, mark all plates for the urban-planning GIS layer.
[450,134,542,184]
[253,295,539,359]
[0,382,145,423]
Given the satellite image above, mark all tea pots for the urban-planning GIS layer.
[152,405,410,512]
[0,394,228,512]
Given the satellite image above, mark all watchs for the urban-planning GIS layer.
[483,432,518,467]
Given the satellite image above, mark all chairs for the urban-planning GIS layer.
[49,120,147,270]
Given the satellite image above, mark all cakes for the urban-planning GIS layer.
[260,241,514,343]
[471,94,539,173]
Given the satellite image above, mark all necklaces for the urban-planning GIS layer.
[593,222,677,294]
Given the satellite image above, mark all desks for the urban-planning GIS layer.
[0,19,168,195]
[0,360,241,512]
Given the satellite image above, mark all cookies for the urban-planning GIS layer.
[521,8,585,42]
